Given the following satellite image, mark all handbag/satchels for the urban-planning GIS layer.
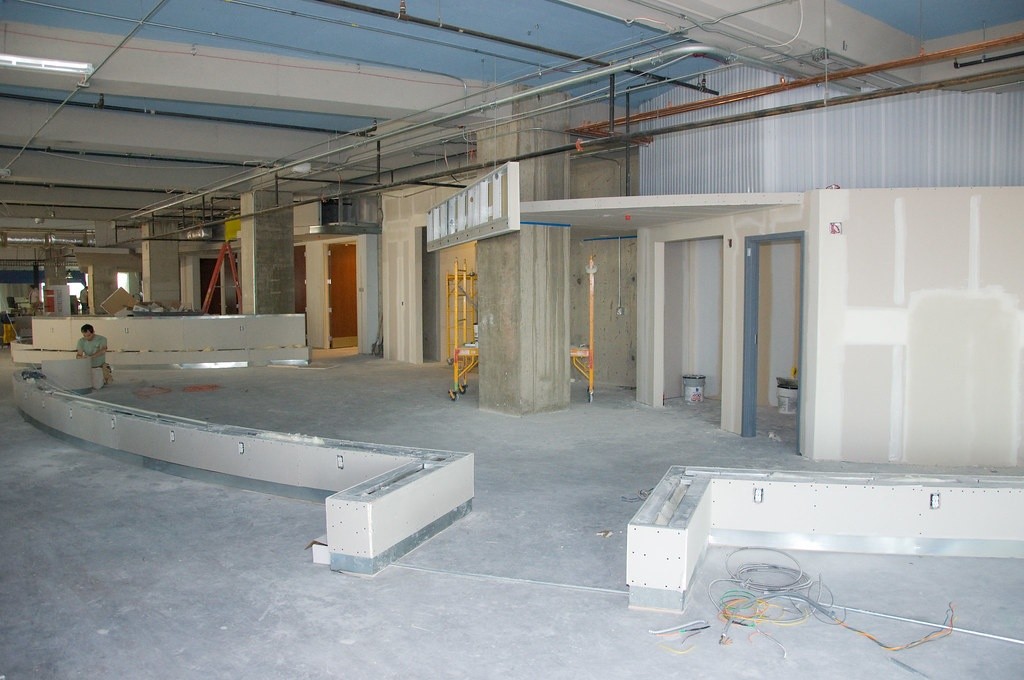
[103,363,113,385]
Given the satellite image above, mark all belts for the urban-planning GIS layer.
[91,366,102,368]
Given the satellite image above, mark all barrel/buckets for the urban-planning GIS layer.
[682,374,706,403]
[776,384,799,415]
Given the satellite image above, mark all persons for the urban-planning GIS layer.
[29,285,39,316]
[80,286,88,314]
[76,324,113,384]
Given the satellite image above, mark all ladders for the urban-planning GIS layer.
[202,241,242,315]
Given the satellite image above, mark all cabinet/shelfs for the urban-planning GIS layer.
[446,254,598,402]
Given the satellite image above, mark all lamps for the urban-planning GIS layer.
[0,53,95,75]
